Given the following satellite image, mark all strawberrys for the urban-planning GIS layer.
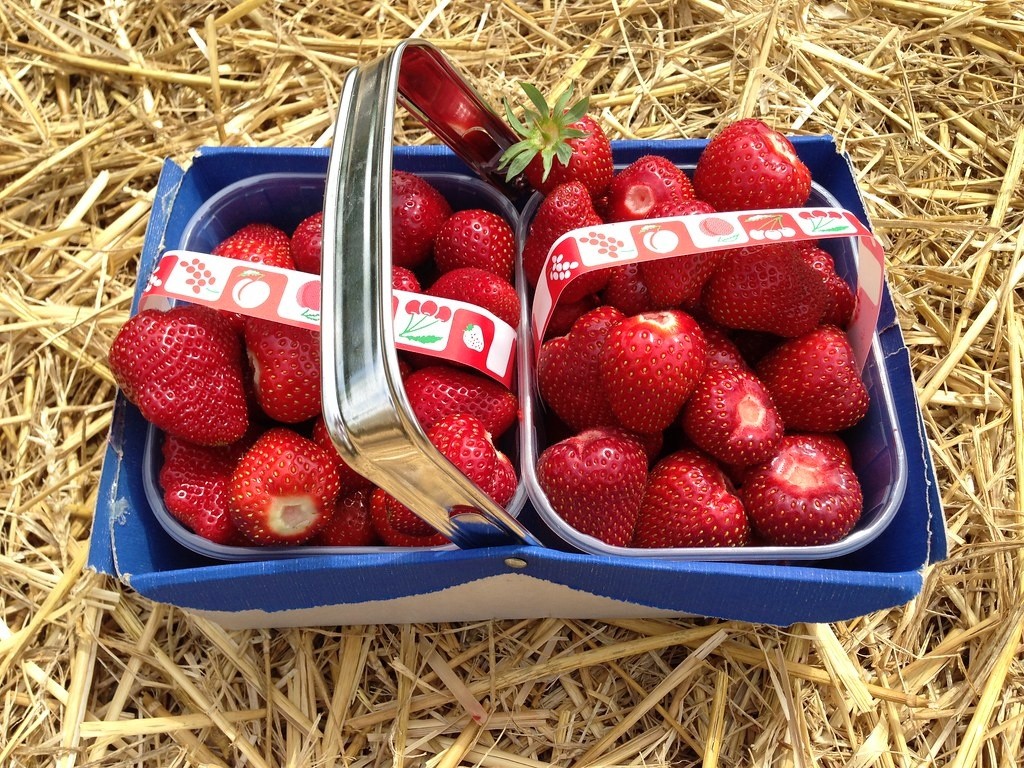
[108,171,522,549]
[497,83,869,550]
